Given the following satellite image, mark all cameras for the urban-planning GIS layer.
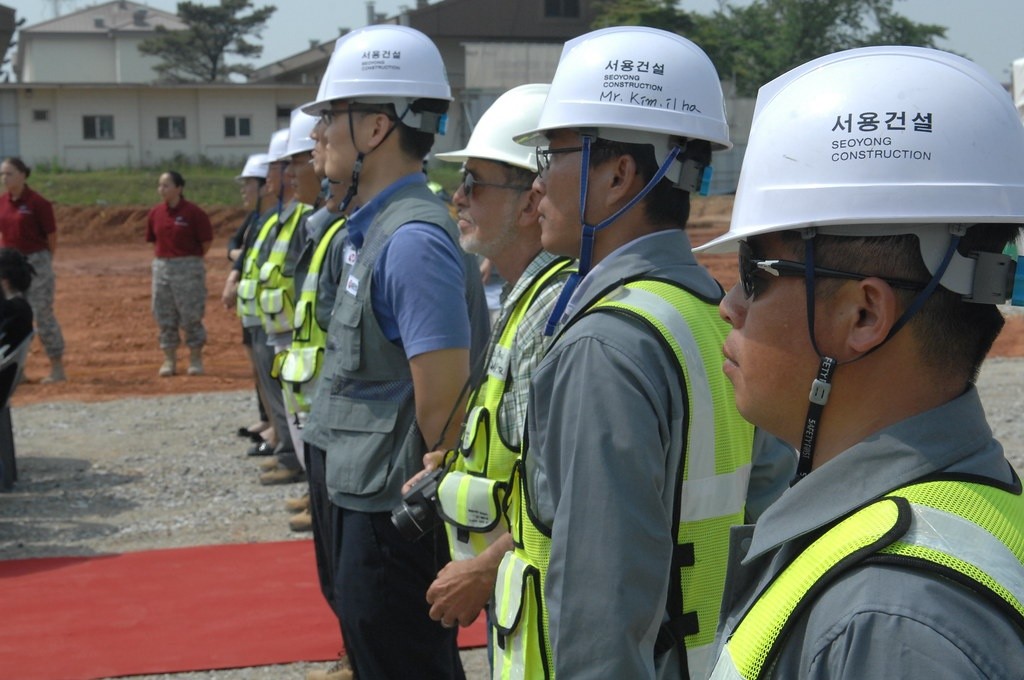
[391,466,450,543]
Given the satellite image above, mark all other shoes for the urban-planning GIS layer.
[247,441,275,456]
[249,434,266,444]
[259,456,283,471]
[260,463,299,485]
[238,426,259,437]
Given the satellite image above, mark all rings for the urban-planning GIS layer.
[440,616,452,628]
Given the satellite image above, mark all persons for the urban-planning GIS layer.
[0,155,69,386]
[223,21,491,680]
[396,22,754,680]
[690,42,1023,680]
[142,169,216,375]
[0,245,37,496]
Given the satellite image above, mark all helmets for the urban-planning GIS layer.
[234,154,270,180]
[263,126,291,164]
[300,23,455,115]
[435,83,559,172]
[277,100,320,160]
[688,45,1024,267]
[513,24,733,158]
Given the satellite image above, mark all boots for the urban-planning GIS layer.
[186,346,204,376]
[40,357,65,384]
[159,349,176,376]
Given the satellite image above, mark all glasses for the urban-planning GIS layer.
[317,108,397,126]
[459,168,533,197]
[736,241,965,301]
[534,141,585,181]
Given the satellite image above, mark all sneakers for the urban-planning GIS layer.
[288,507,313,531]
[306,647,354,680]
[286,490,310,511]
